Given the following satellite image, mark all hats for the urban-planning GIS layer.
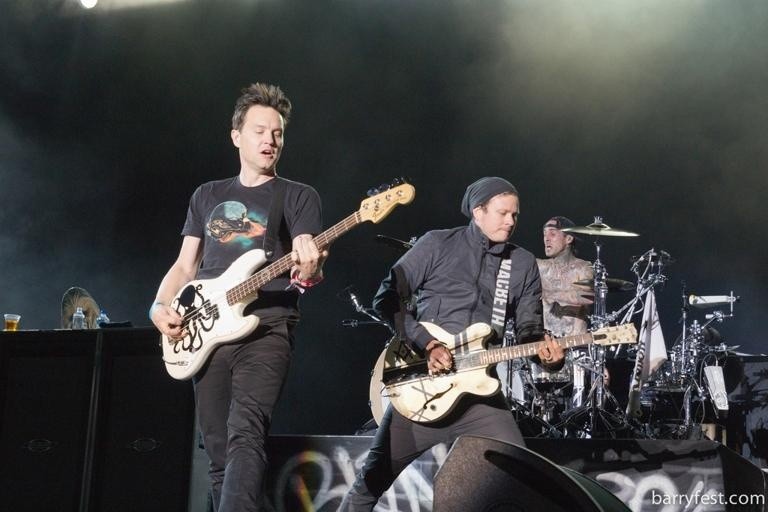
[543,216,577,253]
[461,177,519,219]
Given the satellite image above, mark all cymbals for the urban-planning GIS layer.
[560,223,639,240]
[572,278,633,289]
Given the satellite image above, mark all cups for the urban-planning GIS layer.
[3,313,22,331]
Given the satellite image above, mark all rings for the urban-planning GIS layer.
[543,355,552,361]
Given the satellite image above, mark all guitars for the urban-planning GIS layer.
[161,177,415,380]
[382,322,636,424]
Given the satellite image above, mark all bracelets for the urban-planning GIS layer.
[154,299,166,308]
[289,263,326,288]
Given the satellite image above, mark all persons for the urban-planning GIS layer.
[150,81,333,511]
[534,213,595,336]
[336,175,565,512]
[62,287,102,328]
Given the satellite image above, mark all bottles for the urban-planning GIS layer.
[71,306,86,330]
[95,310,110,328]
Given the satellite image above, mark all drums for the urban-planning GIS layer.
[521,333,572,384]
